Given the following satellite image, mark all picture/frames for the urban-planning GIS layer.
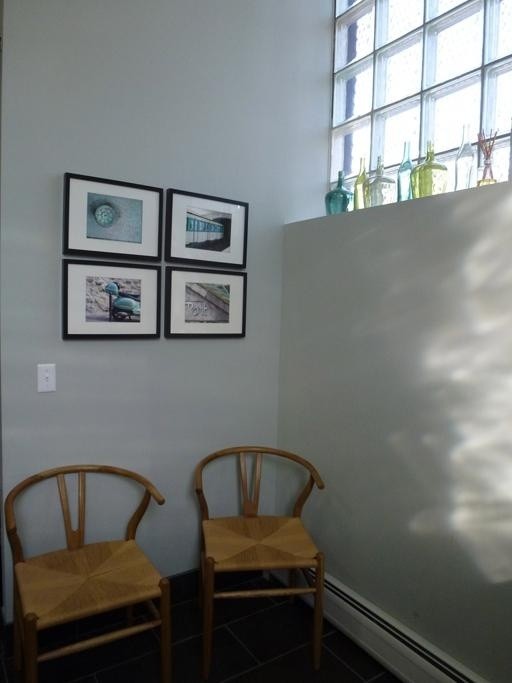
[63,172,163,263]
[164,266,247,338]
[164,188,248,269]
[62,259,161,340]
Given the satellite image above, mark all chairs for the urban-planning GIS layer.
[195,446,325,682]
[1,464,173,683]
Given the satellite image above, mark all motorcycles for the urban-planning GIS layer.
[105,282,141,321]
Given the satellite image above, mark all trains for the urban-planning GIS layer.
[185,211,224,246]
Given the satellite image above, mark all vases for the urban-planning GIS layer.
[325,124,496,216]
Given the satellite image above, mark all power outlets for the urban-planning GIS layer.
[37,363,56,393]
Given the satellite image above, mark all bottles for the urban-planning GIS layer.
[324,124,476,217]
